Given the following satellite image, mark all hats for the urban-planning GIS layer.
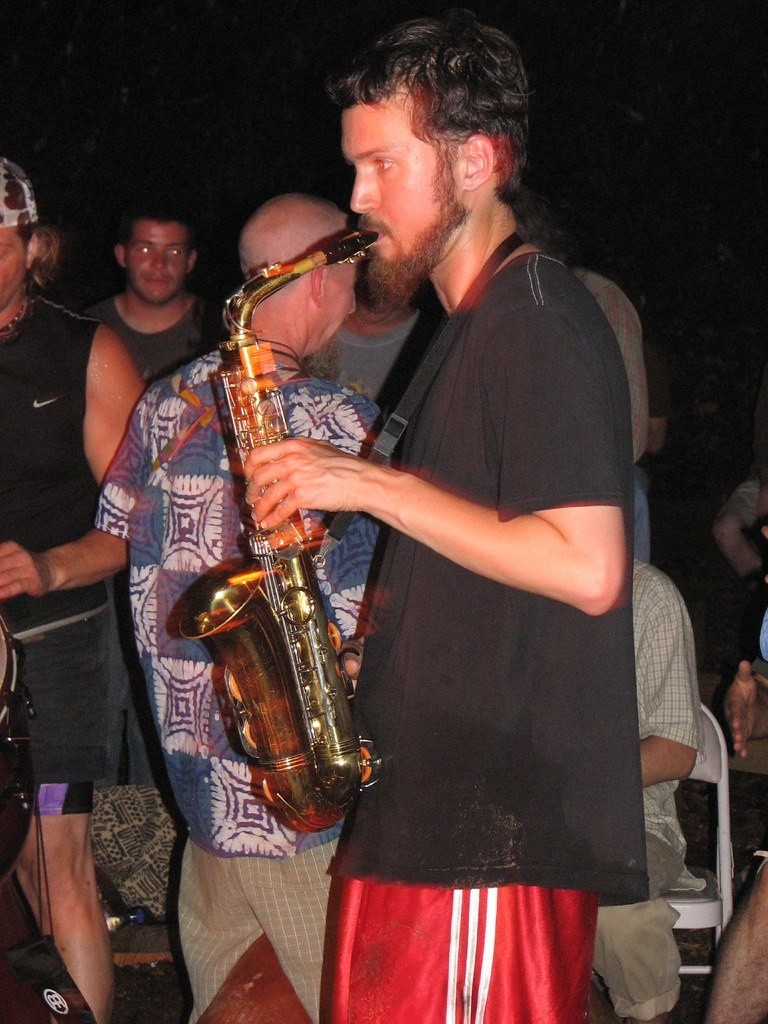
[0,155,38,227]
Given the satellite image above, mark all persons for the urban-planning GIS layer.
[245,11,648,1024]
[647,367,768,1024]
[593,560,705,1024]
[515,187,648,567]
[336,303,420,403]
[84,199,206,788]
[94,194,382,1024]
[0,157,152,1024]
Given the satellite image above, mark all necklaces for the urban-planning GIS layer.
[258,337,301,370]
[0,303,24,334]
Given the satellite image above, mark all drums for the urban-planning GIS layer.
[1,618,36,881]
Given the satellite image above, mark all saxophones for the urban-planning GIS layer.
[176,228,379,826]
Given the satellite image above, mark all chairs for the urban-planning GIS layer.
[664,699,736,976]
